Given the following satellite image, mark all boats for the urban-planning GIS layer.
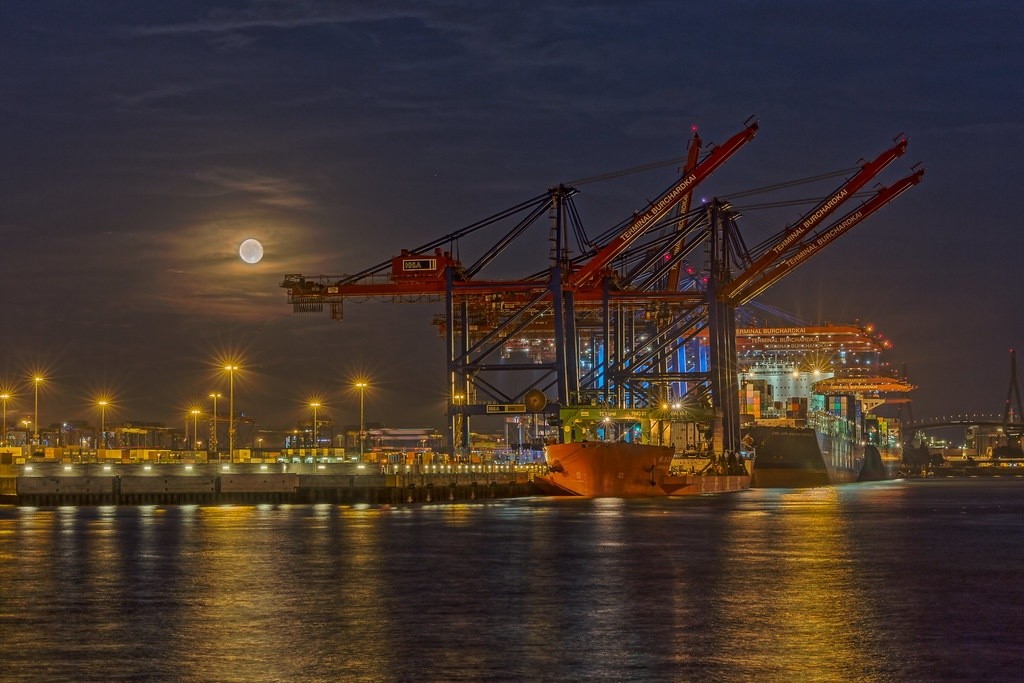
[529,439,758,498]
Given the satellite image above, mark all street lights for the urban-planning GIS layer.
[356,383,367,463]
[455,395,464,447]
[1,395,9,447]
[192,411,199,451]
[224,367,238,463]
[98,401,108,448]
[310,403,321,475]
[210,394,221,459]
[33,377,43,445]
